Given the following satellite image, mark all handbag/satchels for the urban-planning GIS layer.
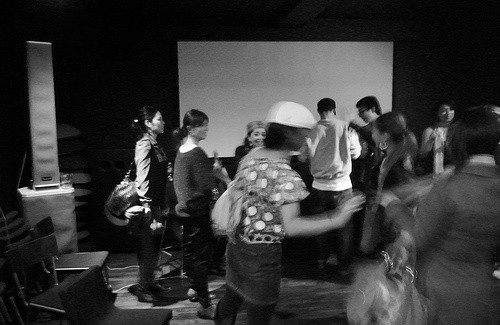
[348,229,426,325]
[210,179,245,237]
[103,159,150,237]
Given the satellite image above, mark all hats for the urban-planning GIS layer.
[264,100,318,131]
[246,120,265,134]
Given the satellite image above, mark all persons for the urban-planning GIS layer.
[296,98,361,282]
[214,99,366,325]
[172,109,226,320]
[128,107,176,303]
[378,103,500,325]
[351,97,456,261]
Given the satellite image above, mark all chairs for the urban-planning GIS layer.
[60,265,175,325]
[35,218,113,285]
[6,237,69,325]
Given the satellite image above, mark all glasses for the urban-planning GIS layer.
[358,106,372,117]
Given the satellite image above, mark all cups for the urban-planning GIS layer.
[61,175,72,188]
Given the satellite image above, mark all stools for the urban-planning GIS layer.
[154,210,204,279]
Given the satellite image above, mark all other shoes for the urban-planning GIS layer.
[186,286,216,320]
[135,275,171,297]
